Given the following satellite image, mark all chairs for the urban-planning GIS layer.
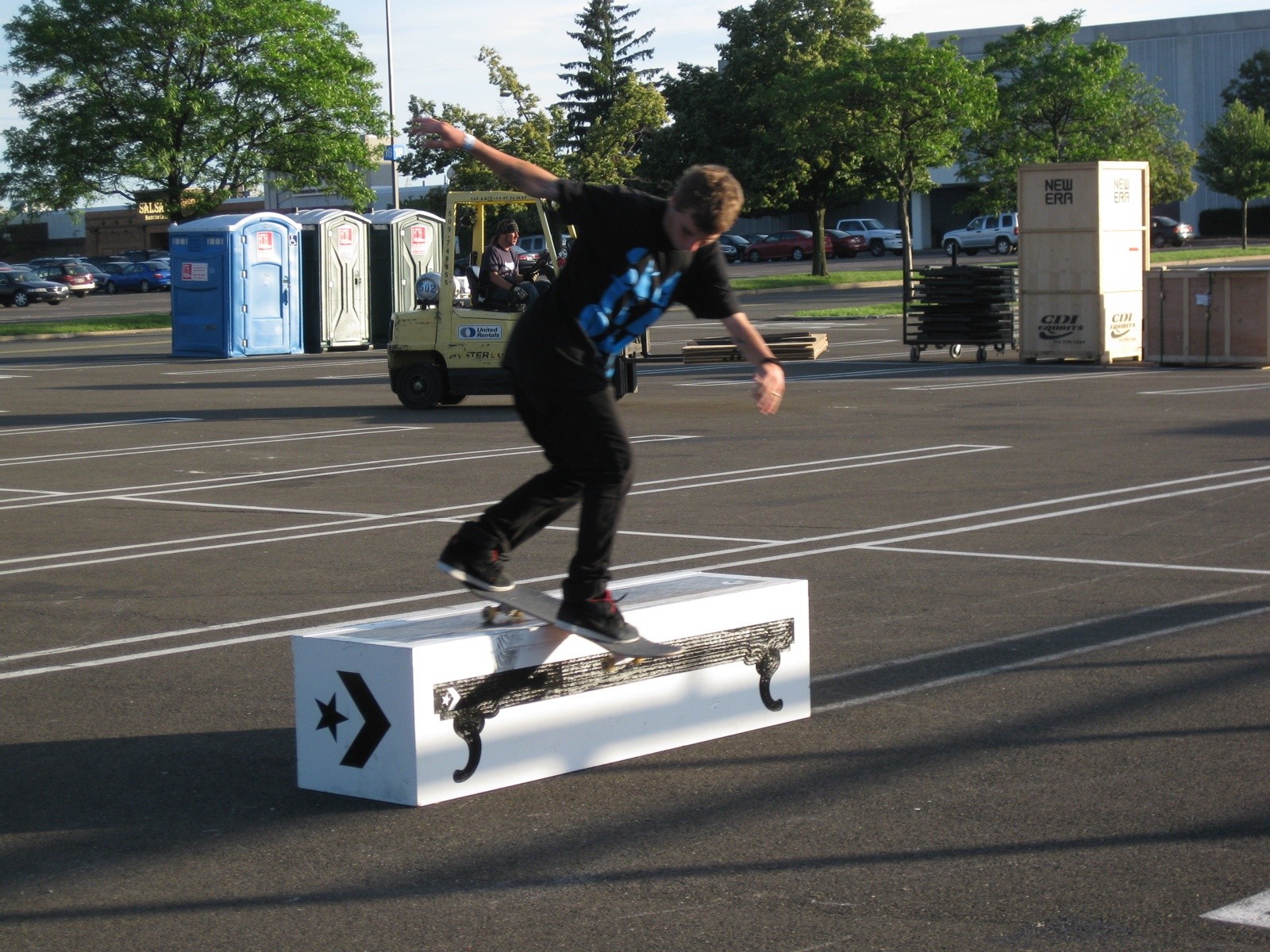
[464,264,485,301]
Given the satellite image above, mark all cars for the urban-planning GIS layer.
[743,229,834,264]
[717,233,779,264]
[0,249,172,307]
[1150,215,1194,248]
[517,233,575,262]
[451,242,541,276]
[813,228,869,260]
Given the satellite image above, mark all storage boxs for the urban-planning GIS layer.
[1143,257,1270,370]
[1014,160,1153,372]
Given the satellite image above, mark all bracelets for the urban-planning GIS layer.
[756,358,786,375]
[510,285,516,292]
[463,134,475,154]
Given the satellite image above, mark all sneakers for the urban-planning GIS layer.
[436,534,516,593]
[553,597,641,643]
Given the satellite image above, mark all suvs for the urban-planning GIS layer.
[941,212,1019,257]
[835,218,904,258]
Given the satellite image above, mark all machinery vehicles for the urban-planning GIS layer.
[383,183,684,412]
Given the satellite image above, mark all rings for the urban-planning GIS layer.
[772,392,780,397]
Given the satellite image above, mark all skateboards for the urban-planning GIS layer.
[465,579,684,672]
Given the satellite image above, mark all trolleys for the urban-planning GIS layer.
[901,268,1016,365]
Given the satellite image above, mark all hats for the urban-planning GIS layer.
[497,219,519,233]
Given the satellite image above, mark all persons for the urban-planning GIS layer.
[411,117,787,644]
[479,219,551,309]
